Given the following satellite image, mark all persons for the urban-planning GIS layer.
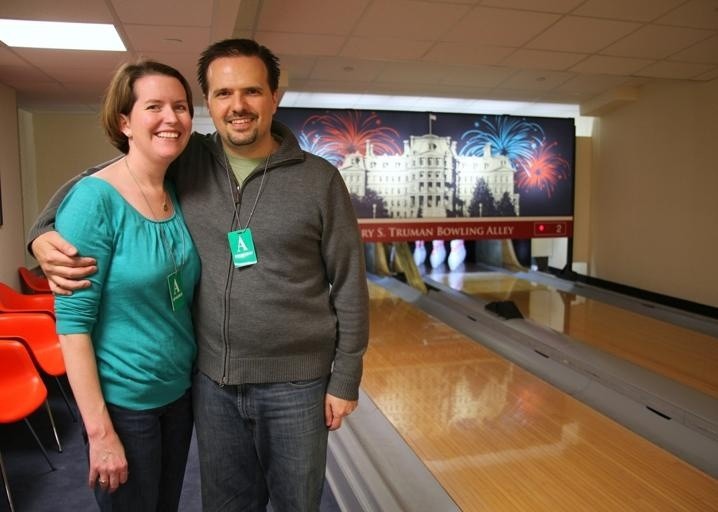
[22,37,371,512]
[51,56,203,511]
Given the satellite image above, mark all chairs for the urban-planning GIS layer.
[19,267,53,294]
[1,312,78,424]
[0,341,59,472]
[0,282,58,322]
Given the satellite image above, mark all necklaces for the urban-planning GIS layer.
[157,194,169,213]
